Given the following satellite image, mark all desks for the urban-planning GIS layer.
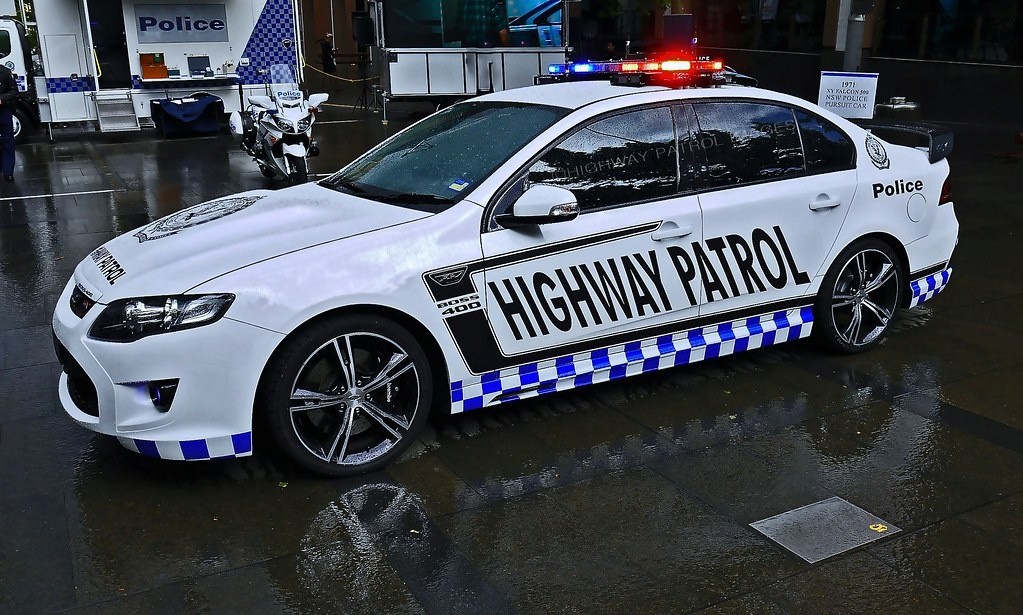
[139,73,245,114]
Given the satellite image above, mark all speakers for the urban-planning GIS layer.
[351,11,374,43]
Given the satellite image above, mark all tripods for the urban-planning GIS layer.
[353,44,384,113]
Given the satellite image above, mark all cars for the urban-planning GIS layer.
[45,54,970,482]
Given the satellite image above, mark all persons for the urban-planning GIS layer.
[320,33,340,90]
[0,64,19,182]
[604,41,619,60]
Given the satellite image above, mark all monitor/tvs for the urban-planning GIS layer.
[187,56,210,77]
[663,14,695,47]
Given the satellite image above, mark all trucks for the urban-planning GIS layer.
[1,0,306,143]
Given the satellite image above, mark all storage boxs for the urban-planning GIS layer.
[149,90,226,138]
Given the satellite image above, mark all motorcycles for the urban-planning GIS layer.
[228,62,330,189]
